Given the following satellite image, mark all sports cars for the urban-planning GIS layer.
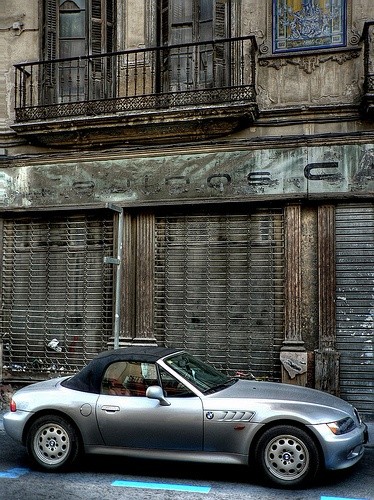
[4,347,369,490]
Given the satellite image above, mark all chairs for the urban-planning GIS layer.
[128,365,147,397]
[109,372,132,396]
